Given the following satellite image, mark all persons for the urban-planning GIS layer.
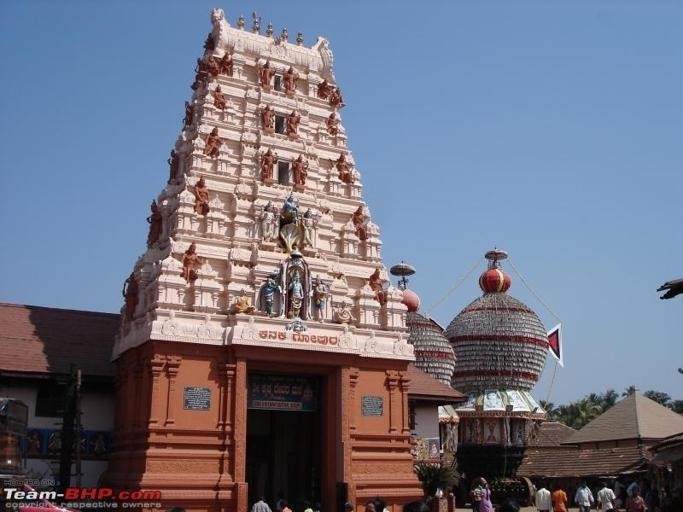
[122,9,390,324]
[166,480,683,512]
[485,390,502,409]
[26,430,107,457]
[431,442,438,457]
